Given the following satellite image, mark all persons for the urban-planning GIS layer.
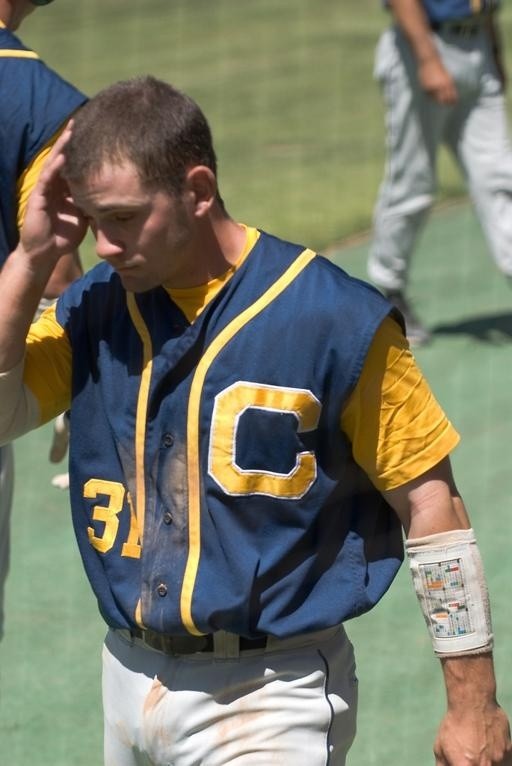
[365,0,512,347]
[0,2,94,656]
[0,75,510,766]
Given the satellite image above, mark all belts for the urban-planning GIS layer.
[120,625,267,657]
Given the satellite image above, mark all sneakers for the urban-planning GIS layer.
[388,289,430,347]
